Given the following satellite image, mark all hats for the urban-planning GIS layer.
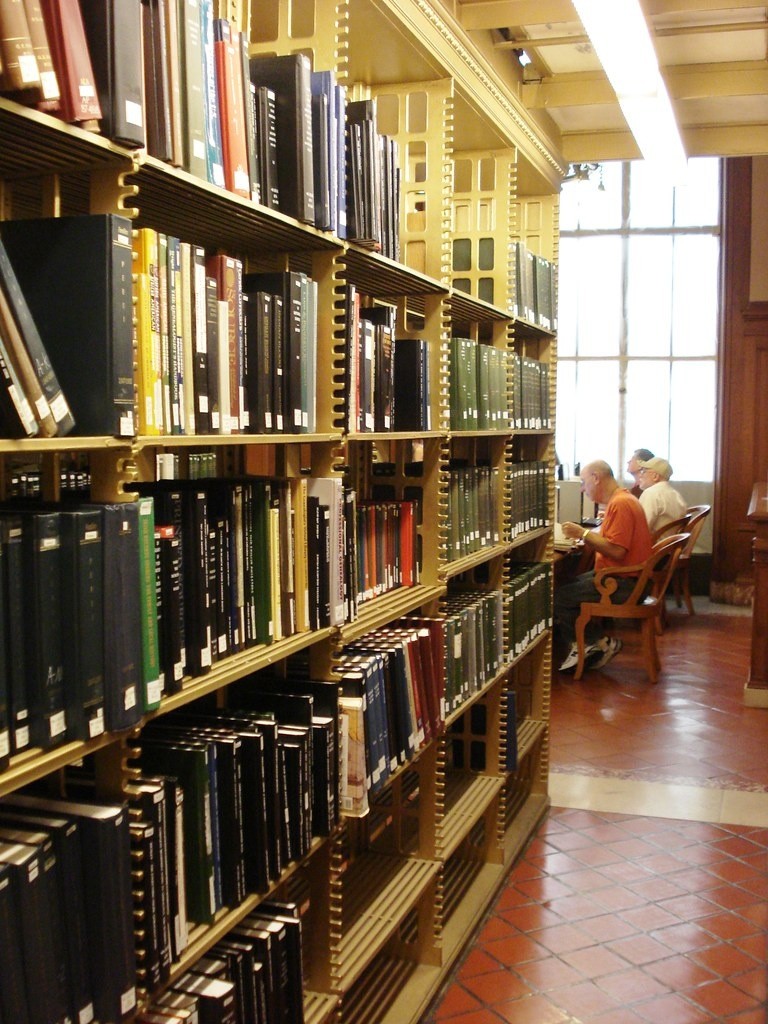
[636,457,673,480]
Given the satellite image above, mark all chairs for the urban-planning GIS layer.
[673,504,712,615]
[573,533,692,683]
[650,512,693,635]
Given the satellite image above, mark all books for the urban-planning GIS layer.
[438,240,554,720]
[1,0,402,260]
[0,215,436,444]
[0,454,443,1024]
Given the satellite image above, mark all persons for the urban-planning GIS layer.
[554,458,654,673]
[599,448,655,521]
[638,456,687,572]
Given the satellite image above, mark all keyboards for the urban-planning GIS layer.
[581,518,602,527]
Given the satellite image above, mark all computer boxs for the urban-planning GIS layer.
[556,480,598,523]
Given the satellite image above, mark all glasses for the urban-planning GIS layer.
[638,468,646,474]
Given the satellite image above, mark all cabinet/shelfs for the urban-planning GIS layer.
[0,0,560,1024]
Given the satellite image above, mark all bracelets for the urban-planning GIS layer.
[581,529,589,541]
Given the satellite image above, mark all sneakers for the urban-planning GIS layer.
[558,636,622,674]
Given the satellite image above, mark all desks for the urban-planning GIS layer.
[742,481,768,710]
[554,509,620,568]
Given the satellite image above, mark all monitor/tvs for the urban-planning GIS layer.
[558,464,570,480]
[574,463,580,476]
